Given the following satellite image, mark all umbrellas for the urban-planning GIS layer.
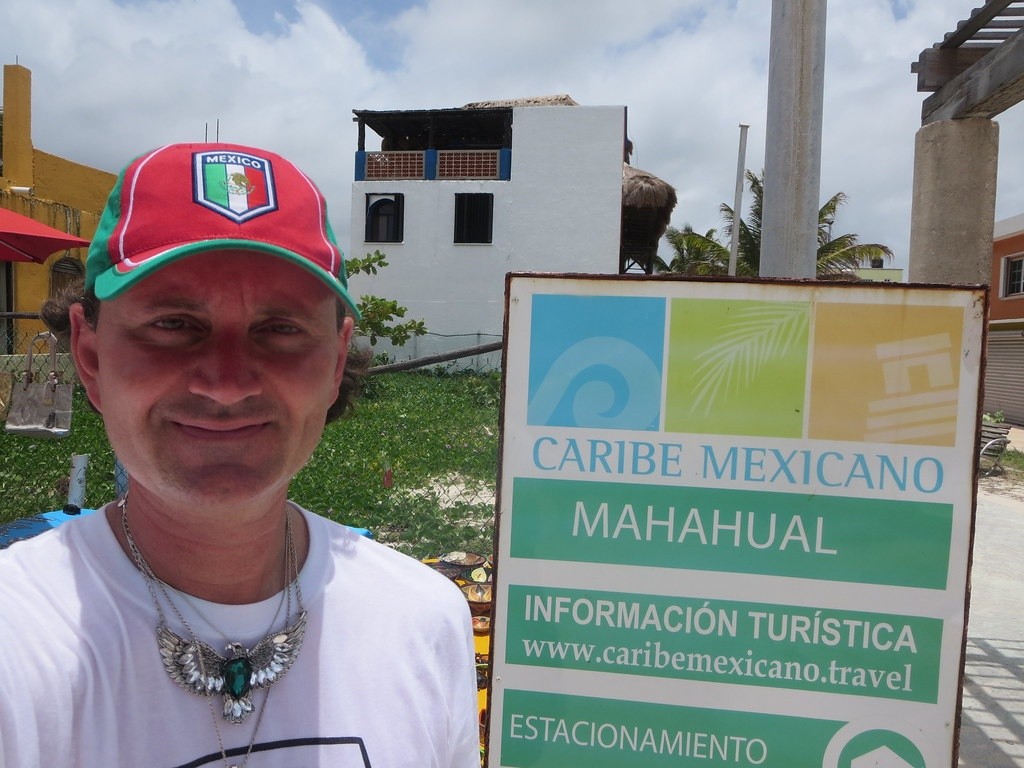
[0,206,91,264]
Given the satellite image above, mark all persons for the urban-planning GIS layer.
[0,143,483,768]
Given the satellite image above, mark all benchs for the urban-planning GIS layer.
[979,421,1012,476]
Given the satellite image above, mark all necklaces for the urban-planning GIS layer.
[118,491,307,768]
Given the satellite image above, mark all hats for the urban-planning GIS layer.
[84,142,363,325]
